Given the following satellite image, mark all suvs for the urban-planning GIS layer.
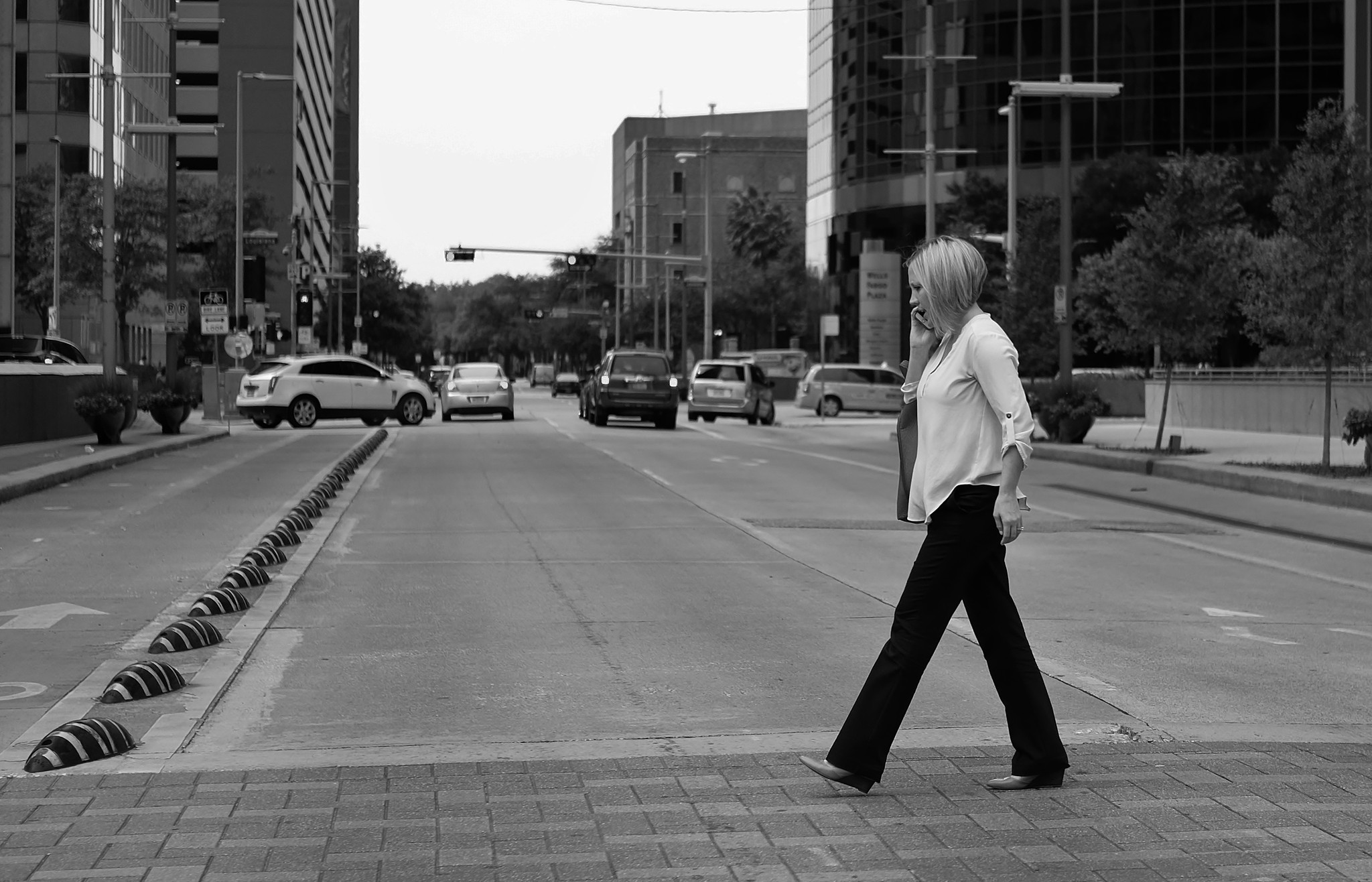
[235,354,437,430]
[579,347,679,429]
[0,335,88,367]
[793,362,906,417]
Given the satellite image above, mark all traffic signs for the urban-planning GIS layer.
[164,299,188,326]
[201,315,229,335]
[569,264,592,271]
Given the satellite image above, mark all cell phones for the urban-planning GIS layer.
[913,311,934,330]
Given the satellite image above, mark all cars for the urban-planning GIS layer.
[436,361,516,422]
[382,364,452,398]
[549,371,581,397]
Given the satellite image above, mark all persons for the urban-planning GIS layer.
[797,239,1069,790]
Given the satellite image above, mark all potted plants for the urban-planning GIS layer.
[74,368,137,445]
[1028,372,1112,443]
[136,361,202,435]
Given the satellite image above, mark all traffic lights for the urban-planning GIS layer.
[566,255,597,266]
[524,310,545,319]
[445,251,475,262]
[296,288,313,327]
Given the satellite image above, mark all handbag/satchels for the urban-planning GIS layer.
[897,401,926,525]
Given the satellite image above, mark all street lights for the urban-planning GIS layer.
[673,149,712,361]
[308,178,368,358]
[235,70,298,327]
[49,134,63,339]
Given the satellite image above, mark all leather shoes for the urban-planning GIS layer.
[800,756,875,794]
[987,771,1064,789]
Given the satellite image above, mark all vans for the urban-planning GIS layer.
[719,348,811,378]
[529,363,555,388]
[687,359,776,426]
